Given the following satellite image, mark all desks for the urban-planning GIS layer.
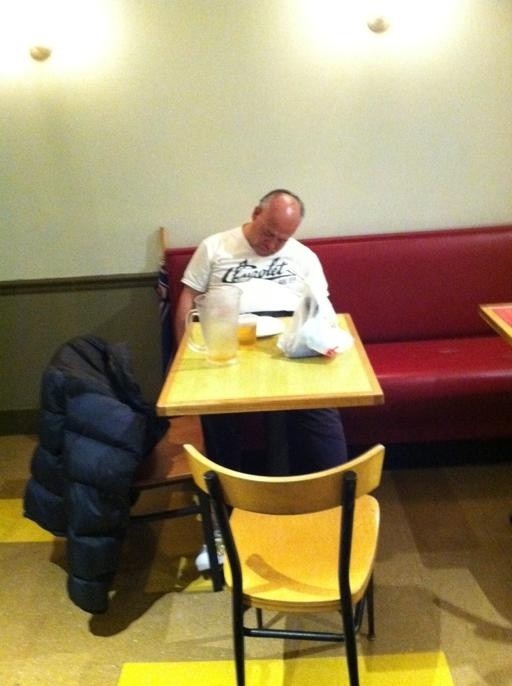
[476,302,511,344]
[156,312,384,591]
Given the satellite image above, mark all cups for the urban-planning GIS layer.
[236,313,258,349]
[182,286,244,366]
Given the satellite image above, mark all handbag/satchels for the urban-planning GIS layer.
[276,285,358,361]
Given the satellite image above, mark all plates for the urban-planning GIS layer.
[255,314,286,338]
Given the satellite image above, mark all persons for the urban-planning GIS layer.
[172,188,347,576]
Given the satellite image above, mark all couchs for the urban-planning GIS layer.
[165,224,512,465]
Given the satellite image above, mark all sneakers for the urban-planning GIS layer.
[193,531,226,573]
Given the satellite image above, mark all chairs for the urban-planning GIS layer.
[130,414,226,590]
[181,440,385,686]
